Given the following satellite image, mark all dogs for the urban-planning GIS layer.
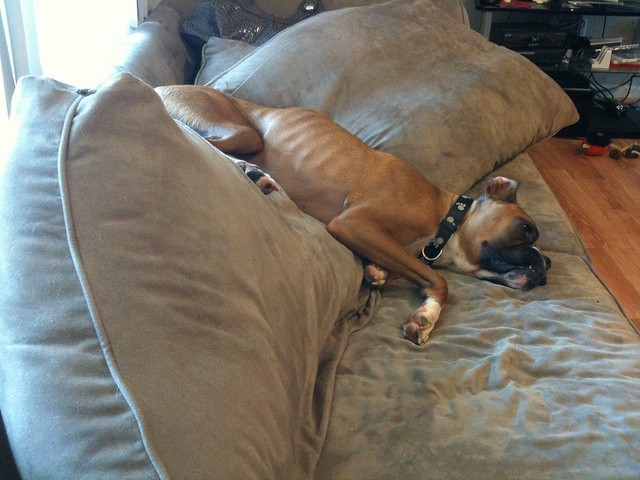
[152,85,552,345]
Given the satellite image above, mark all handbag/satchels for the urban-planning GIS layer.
[179,0,329,60]
